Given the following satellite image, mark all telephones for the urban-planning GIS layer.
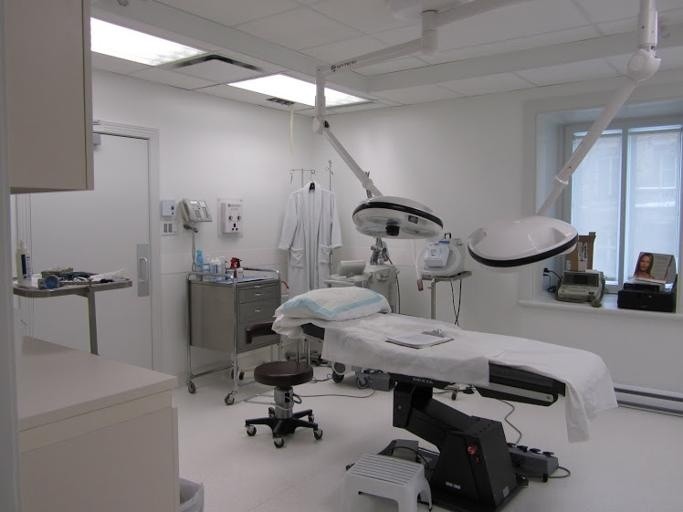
[179,199,213,222]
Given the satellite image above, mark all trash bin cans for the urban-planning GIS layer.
[178,479,203,511]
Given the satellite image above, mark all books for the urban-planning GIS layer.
[384,332,452,349]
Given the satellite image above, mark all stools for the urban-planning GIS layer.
[338,452,432,512]
[243,358,324,449]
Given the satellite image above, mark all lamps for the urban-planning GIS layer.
[314,0,663,270]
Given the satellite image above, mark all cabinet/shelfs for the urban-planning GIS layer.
[0,0,93,196]
[186,277,280,405]
[14,333,180,512]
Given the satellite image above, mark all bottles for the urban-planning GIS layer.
[15,240,33,283]
[195,250,240,283]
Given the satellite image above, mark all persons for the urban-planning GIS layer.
[633,253,656,279]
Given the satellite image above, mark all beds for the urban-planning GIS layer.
[278,282,604,512]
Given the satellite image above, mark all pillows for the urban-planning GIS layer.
[280,285,389,322]
